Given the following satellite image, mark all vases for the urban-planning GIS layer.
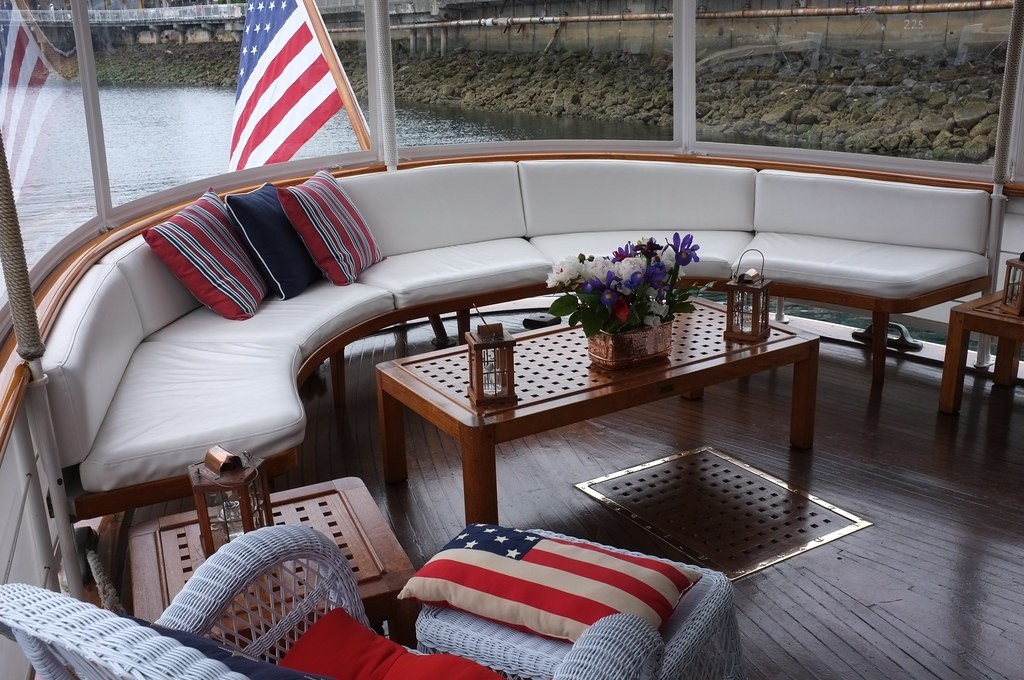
[588,314,675,370]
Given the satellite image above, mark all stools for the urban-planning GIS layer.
[415,529,748,680]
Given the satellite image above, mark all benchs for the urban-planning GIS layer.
[22,152,1006,523]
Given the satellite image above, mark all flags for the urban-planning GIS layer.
[227,0,344,174]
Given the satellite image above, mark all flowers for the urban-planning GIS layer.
[546,232,701,339]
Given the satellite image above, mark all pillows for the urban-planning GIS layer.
[140,186,269,321]
[225,182,327,301]
[121,612,333,680]
[276,609,507,680]
[397,523,703,644]
[278,166,386,286]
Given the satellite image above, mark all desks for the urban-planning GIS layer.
[937,289,1024,415]
[375,294,820,525]
[130,476,422,665]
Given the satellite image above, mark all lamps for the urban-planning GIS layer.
[189,445,275,559]
[724,248,773,343]
[466,303,518,406]
[999,251,1024,317]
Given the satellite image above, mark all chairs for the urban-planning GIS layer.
[1,524,665,680]
